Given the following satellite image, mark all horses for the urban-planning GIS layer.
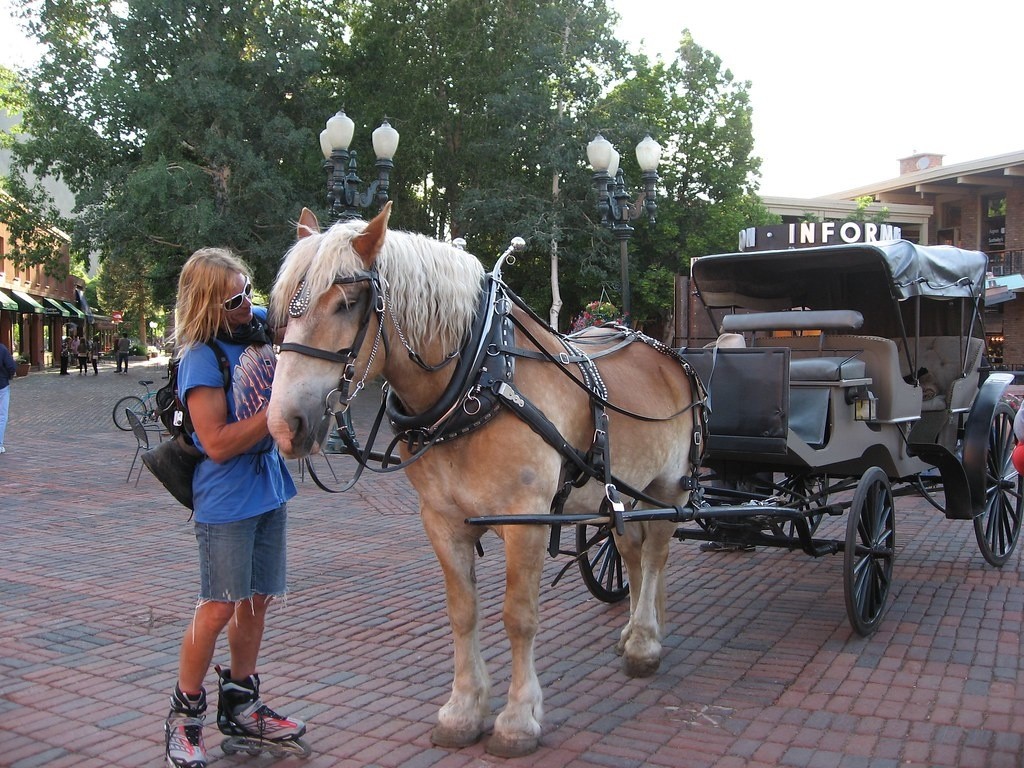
[264,201,710,757]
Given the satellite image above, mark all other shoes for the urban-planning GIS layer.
[124,371,127,375]
[0,446,5,454]
[114,370,122,373]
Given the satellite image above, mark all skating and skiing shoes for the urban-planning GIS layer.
[164,680,208,768]
[214,664,311,760]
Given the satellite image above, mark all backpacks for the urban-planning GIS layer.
[155,313,275,447]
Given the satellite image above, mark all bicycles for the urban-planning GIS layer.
[112,376,169,432]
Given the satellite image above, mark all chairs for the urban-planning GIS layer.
[123,407,171,489]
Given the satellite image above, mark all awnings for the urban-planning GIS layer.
[62,301,85,319]
[12,290,44,314]
[76,289,95,325]
[43,297,70,317]
[0,290,19,311]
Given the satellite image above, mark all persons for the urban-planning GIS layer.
[0,343,18,453]
[113,332,131,374]
[60,335,100,375]
[162,246,314,768]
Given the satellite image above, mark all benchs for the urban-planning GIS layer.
[741,336,923,425]
[789,355,874,446]
[889,335,985,413]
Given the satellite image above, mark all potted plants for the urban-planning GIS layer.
[15,356,31,377]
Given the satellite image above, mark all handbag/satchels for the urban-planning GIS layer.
[93,355,98,359]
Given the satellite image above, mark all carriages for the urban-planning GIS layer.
[261,197,1024,761]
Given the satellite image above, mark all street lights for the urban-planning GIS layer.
[586,130,663,330]
[314,110,400,456]
[149,321,158,346]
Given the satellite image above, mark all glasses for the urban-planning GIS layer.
[207,275,252,311]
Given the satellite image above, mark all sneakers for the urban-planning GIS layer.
[141,429,205,522]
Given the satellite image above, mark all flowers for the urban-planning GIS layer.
[567,300,620,336]
